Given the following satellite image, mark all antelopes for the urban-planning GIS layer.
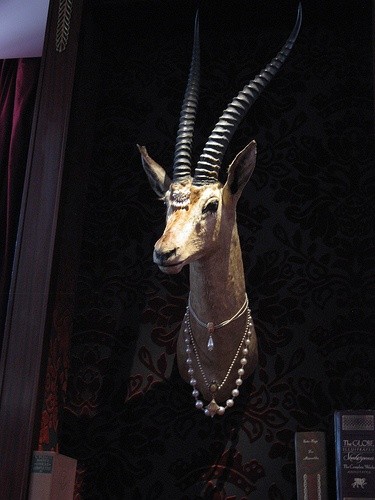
[137,2,303,402]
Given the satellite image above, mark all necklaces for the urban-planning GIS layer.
[187,293,249,351]
[185,307,252,394]
[180,309,252,418]
